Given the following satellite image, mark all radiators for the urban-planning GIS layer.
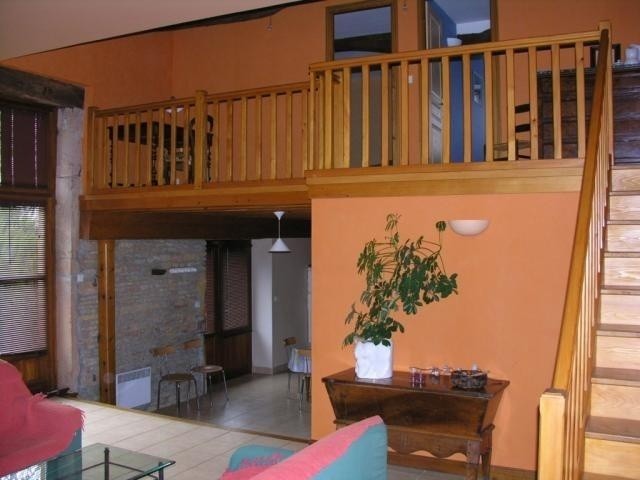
[116,364,152,410]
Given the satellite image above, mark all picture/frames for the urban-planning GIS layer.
[589,42,622,67]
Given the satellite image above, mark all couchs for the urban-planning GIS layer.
[216,414,388,480]
[0,361,85,480]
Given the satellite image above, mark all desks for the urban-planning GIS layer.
[319,365,511,480]
[104,117,213,187]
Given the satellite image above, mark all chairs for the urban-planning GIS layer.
[180,339,231,407]
[282,336,303,401]
[148,345,201,417]
[161,113,215,183]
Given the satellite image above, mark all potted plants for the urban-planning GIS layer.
[340,212,461,381]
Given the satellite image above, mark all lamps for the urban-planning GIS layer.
[268,211,292,253]
[446,219,488,237]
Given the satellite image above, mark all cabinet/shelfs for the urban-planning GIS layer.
[535,62,639,163]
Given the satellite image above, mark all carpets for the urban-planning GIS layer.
[43,395,317,480]
